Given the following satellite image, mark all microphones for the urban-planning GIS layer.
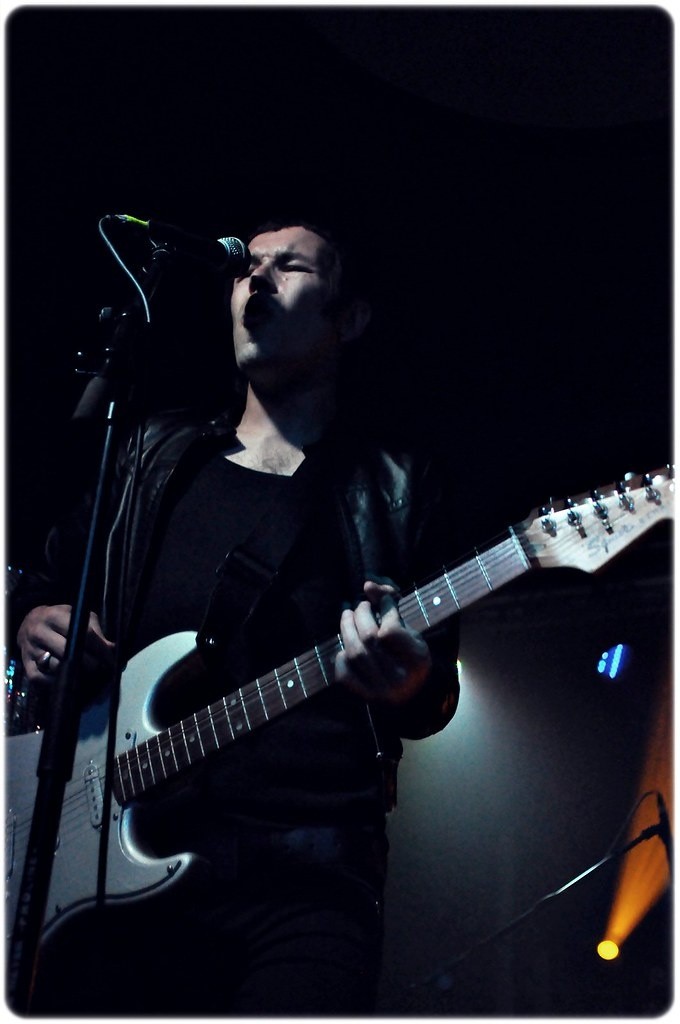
[121,215,246,277]
[656,792,672,864]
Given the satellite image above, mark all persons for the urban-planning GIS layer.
[7,218,460,1016]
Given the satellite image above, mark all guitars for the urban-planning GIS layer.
[4,458,674,980]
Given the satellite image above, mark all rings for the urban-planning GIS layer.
[36,652,50,666]
